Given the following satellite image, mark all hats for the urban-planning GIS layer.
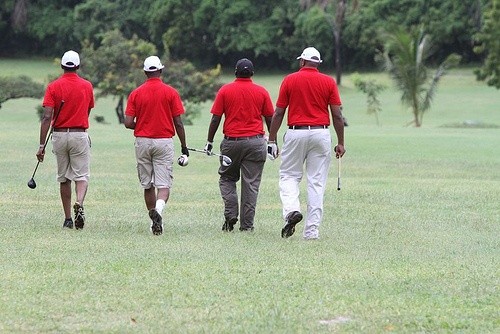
[234,58,254,78]
[61,50,81,68]
[297,46,323,64]
[143,55,164,73]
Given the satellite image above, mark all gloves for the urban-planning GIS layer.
[204,142,214,156]
[266,141,278,160]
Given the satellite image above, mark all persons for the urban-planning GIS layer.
[36,51,95,230]
[267,47,345,241]
[125,56,189,234]
[204,59,279,231]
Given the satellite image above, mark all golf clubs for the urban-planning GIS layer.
[27,99,65,189]
[334,145,341,190]
[186,147,233,167]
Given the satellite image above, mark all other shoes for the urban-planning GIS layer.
[280,211,303,239]
[221,216,255,233]
[147,208,164,235]
[61,202,86,231]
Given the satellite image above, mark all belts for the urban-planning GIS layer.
[289,125,328,129]
[53,127,85,132]
[224,134,263,141]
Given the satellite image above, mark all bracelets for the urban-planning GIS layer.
[39,145,45,148]
